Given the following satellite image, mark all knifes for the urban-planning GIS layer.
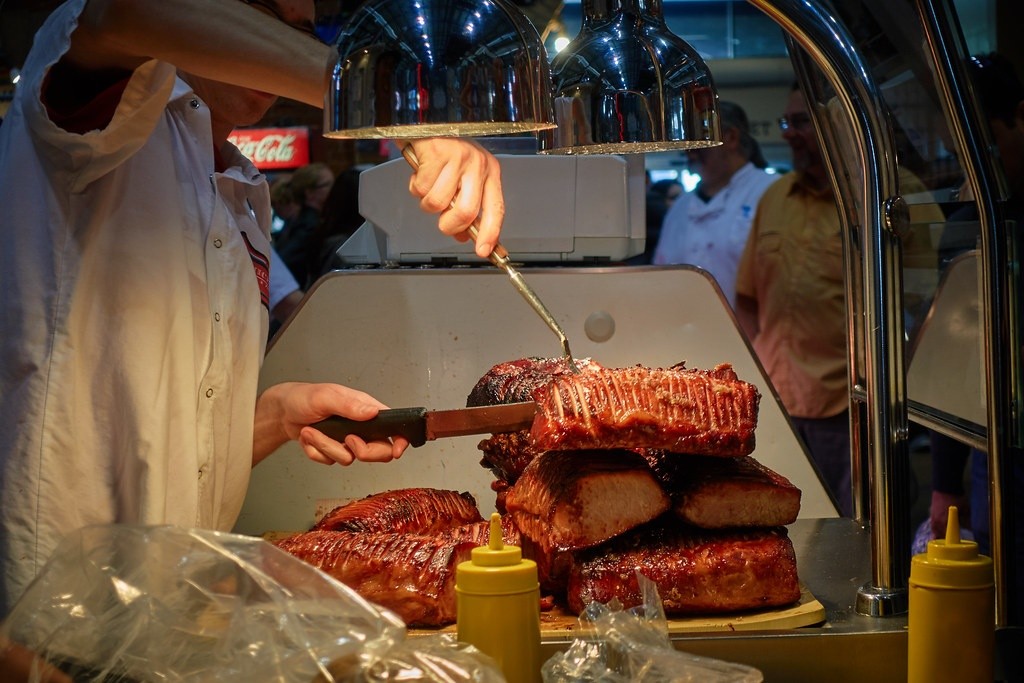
[306,400,536,449]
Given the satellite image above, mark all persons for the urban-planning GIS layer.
[276,165,337,245]
[733,84,947,518]
[269,246,305,323]
[0,0,507,683]
[652,179,687,207]
[652,101,782,306]
[930,180,1024,545]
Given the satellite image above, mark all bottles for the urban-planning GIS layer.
[453,513,543,683]
[907,506,994,683]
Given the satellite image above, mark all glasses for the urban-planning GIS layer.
[779,114,813,129]
[247,0,322,42]
